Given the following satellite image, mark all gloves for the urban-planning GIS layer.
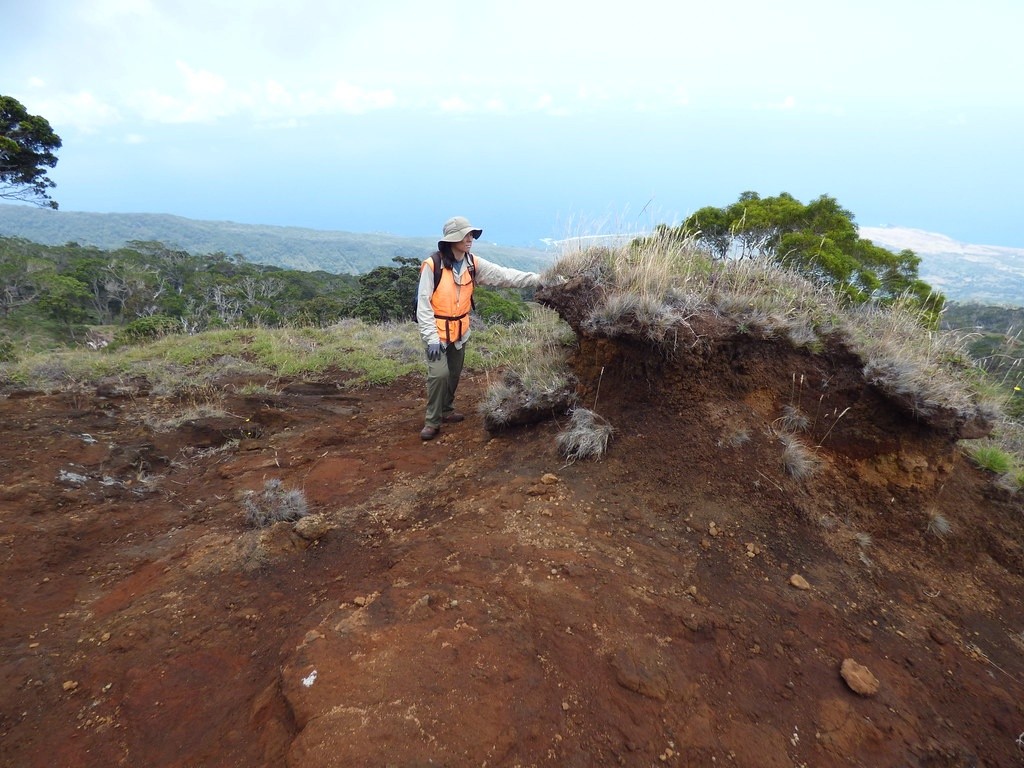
[427,343,446,362]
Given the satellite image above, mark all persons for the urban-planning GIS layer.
[416,217,567,439]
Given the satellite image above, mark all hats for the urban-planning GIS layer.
[438,217,482,253]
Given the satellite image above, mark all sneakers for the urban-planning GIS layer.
[421,425,439,439]
[442,411,465,423]
